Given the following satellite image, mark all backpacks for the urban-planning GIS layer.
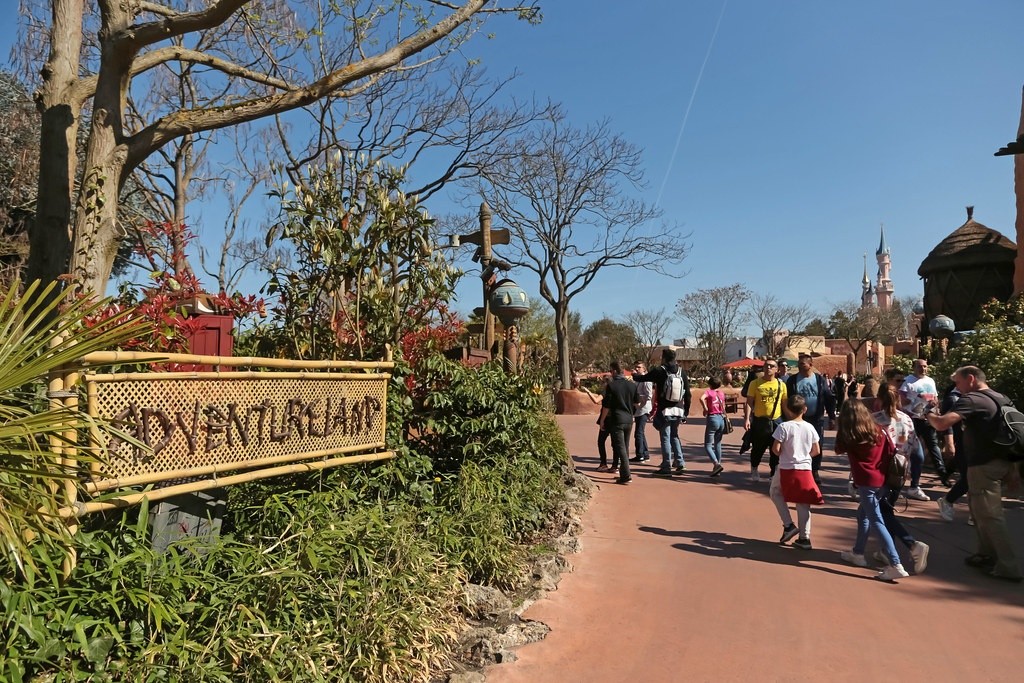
[881,426,909,512]
[661,365,684,402]
[962,390,1024,461]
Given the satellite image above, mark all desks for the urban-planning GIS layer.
[725,402,746,414]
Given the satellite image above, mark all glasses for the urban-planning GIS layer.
[780,364,788,367]
[764,364,775,368]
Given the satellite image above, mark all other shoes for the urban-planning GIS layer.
[848,481,857,499]
[710,464,723,477]
[968,516,975,525]
[673,466,688,473]
[941,473,953,487]
[813,470,822,484]
[596,457,650,473]
[651,469,672,477]
[615,478,632,485]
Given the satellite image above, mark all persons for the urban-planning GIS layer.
[868,383,929,576]
[632,349,692,477]
[581,375,619,474]
[628,360,652,464]
[647,383,686,466]
[770,395,824,550]
[739,352,1024,584]
[834,399,910,581]
[700,376,727,477]
[601,360,641,484]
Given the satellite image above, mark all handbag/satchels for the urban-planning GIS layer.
[723,417,733,434]
[749,416,773,441]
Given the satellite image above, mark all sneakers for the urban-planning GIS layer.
[780,521,799,544]
[751,465,760,481]
[937,497,954,521]
[878,564,910,580]
[872,551,890,564]
[907,486,930,500]
[910,540,929,574]
[792,539,812,550]
[840,551,867,566]
